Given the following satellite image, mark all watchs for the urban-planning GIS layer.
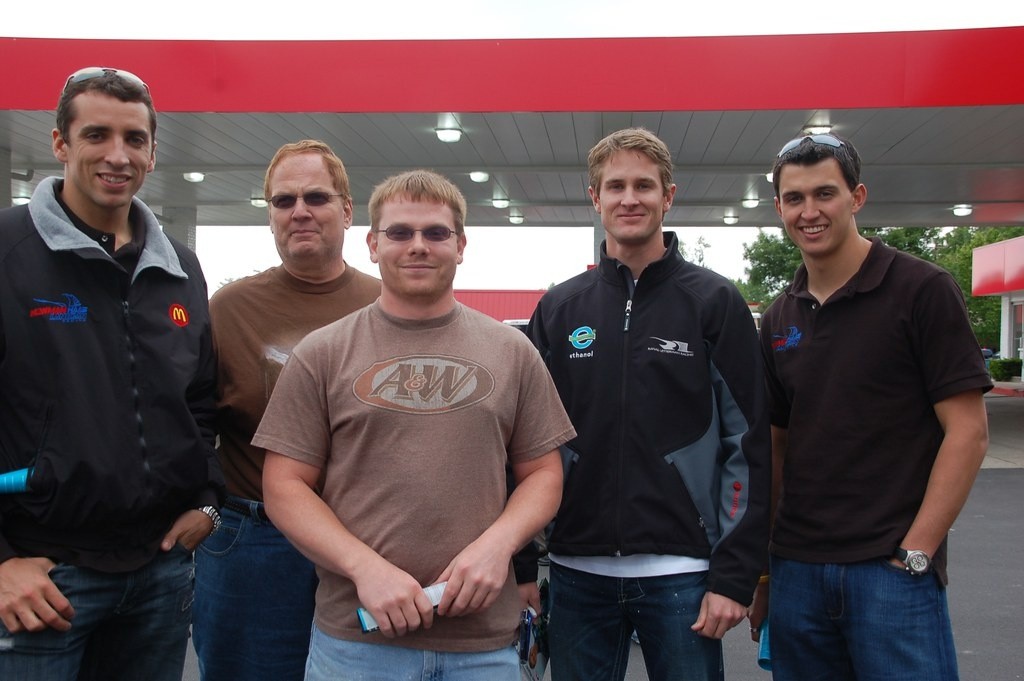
[892,547,933,575]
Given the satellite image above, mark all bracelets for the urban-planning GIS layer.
[197,506,222,536]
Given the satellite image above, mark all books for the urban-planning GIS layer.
[512,577,551,680]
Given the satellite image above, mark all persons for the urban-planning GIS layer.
[193,136,384,681]
[758,130,994,681]
[251,168,577,680]
[522,127,773,680]
[0,66,226,681]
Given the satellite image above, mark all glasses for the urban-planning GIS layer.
[375,225,455,241]
[61,66,150,96]
[776,133,852,160]
[266,191,344,210]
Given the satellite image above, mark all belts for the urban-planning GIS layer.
[222,500,270,520]
